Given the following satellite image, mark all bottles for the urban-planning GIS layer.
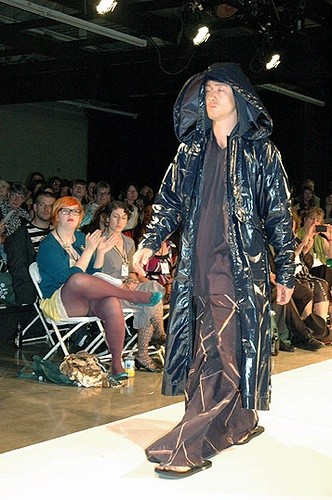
[124,352,136,378]
[271,328,279,356]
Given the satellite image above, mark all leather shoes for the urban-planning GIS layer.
[298,336,325,351]
[281,343,297,351]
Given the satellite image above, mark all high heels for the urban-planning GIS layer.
[149,337,166,348]
[134,358,161,373]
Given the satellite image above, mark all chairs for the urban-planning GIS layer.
[15,262,171,373]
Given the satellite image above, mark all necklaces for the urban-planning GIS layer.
[56,228,79,261]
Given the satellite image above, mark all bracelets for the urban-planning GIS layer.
[328,239,332,241]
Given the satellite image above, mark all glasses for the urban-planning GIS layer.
[12,194,24,200]
[53,182,61,187]
[59,208,81,216]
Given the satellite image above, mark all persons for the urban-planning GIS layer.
[0,172,332,382]
[132,64,301,479]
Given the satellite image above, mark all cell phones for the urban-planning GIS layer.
[316,226,327,232]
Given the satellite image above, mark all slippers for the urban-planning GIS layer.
[234,425,265,445]
[155,459,212,479]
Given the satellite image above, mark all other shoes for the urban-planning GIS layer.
[114,372,128,380]
[132,291,162,307]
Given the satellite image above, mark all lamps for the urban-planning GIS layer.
[243,30,281,73]
[160,20,212,46]
